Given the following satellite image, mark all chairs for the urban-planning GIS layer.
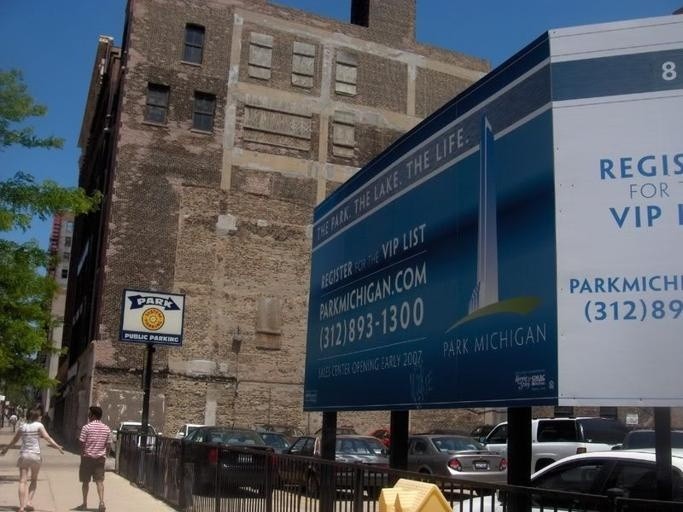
[212,433,255,444]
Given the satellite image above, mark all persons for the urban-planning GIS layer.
[39,411,51,437]
[70,405,116,511]
[18,413,26,425]
[0,408,65,512]
[8,411,16,431]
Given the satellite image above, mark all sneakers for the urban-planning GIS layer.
[99,504,106,511]
[75,504,87,511]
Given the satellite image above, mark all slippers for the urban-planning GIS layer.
[18,505,34,511]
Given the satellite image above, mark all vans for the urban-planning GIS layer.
[479,416,627,475]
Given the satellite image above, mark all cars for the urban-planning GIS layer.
[451,426,683,512]
[168,421,389,498]
[109,422,163,459]
[409,434,508,496]
